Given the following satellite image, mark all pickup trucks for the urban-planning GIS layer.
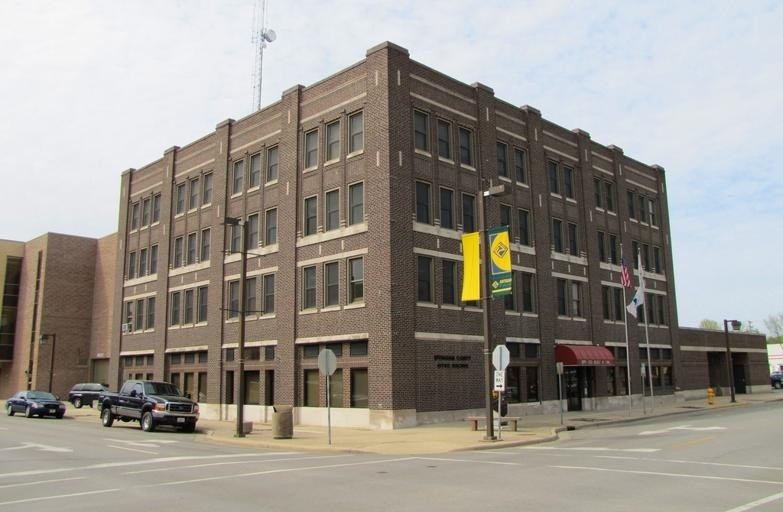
[98,379,200,433]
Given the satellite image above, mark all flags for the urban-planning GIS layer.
[620,249,632,289]
[625,253,645,319]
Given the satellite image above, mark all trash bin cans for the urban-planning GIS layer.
[272,405,293,439]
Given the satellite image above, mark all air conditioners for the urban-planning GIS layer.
[122,324,132,332]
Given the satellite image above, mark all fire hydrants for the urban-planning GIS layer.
[707,388,715,405]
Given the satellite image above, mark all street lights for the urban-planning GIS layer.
[478,184,513,441]
[40,333,57,392]
[220,216,250,439]
[723,319,740,403]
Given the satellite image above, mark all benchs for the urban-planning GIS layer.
[465,416,520,431]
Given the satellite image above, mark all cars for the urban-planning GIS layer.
[68,382,112,409]
[770,370,783,390]
[6,390,66,419]
